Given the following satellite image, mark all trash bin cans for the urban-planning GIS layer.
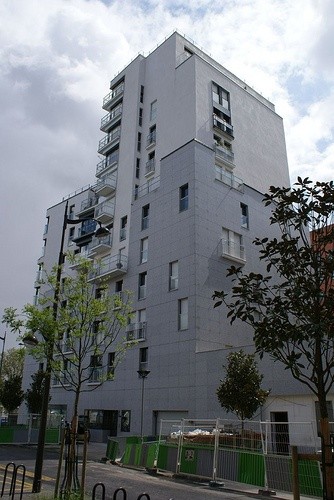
[104,439,120,463]
